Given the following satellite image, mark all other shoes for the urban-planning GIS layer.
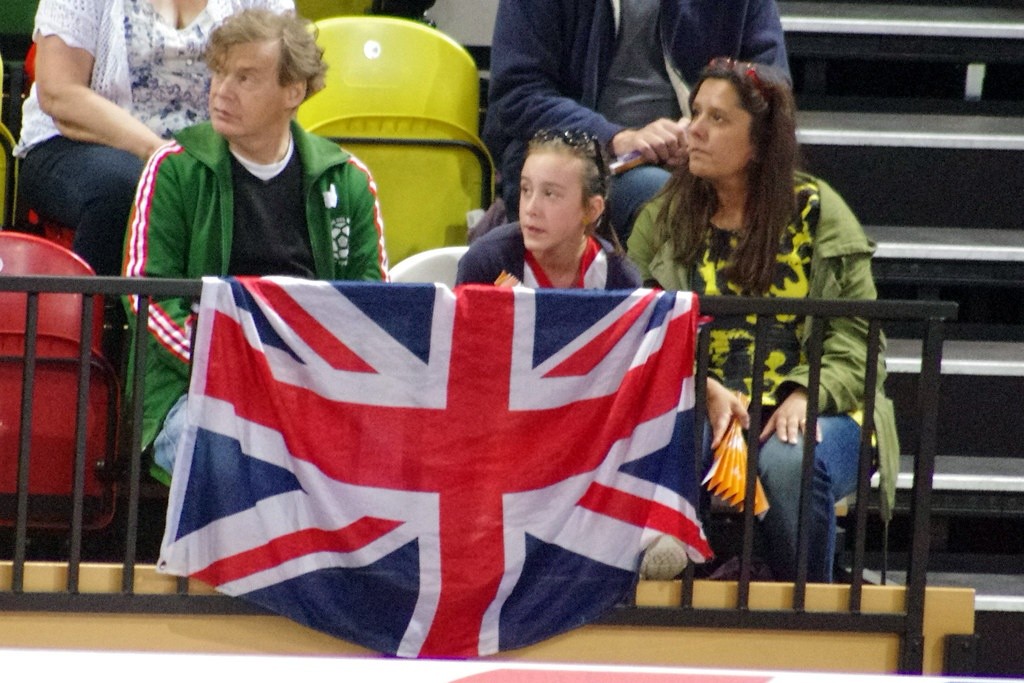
[638,537,687,581]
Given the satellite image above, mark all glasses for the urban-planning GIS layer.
[532,127,605,183]
[710,57,763,93]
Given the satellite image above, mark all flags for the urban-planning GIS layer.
[154,276,715,659]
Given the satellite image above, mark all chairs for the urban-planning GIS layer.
[291,0,375,22]
[422,0,500,116]
[386,246,473,290]
[24,43,76,251]
[291,15,497,268]
[0,231,121,535]
[0,52,19,228]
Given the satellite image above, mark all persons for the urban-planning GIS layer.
[623,57,899,584]
[12,0,298,360]
[120,5,387,479]
[486,0,793,248]
[456,126,687,580]
